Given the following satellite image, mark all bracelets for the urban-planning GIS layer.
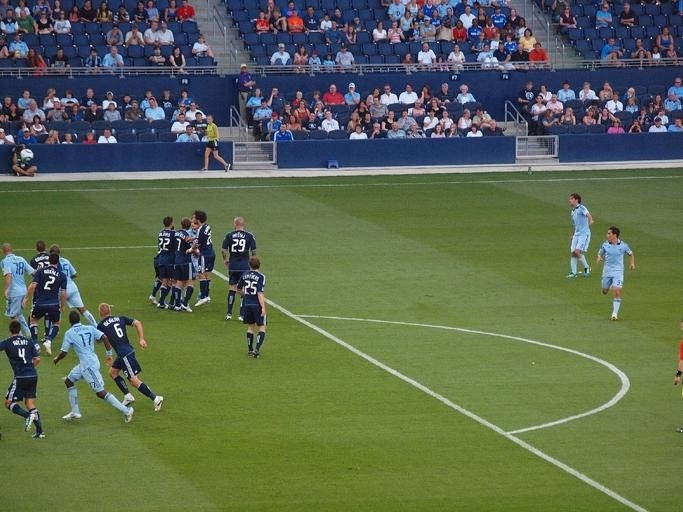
[676,370,682,377]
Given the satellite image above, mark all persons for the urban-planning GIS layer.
[674,320,683,432]
[1,0,683,145]
[597,227,634,320]
[1,240,166,439]
[12,144,37,177]
[566,192,592,278]
[150,210,267,361]
[200,114,231,172]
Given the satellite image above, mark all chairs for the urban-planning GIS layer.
[533,84,683,133]
[0,95,205,143]
[248,90,505,139]
[0,0,218,73]
[537,0,683,58]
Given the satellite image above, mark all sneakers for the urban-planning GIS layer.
[200,168,207,171]
[62,412,82,419]
[32,431,46,439]
[25,414,35,431]
[238,316,243,321]
[153,395,163,412]
[566,272,578,279]
[609,315,618,321]
[149,294,210,313]
[247,350,262,358]
[583,266,592,278]
[225,163,231,173]
[224,313,232,320]
[34,338,52,356]
[122,392,135,423]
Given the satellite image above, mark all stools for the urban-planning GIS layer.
[327,160,339,168]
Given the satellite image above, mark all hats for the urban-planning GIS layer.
[271,112,278,117]
[240,63,247,69]
[349,82,355,89]
[23,128,29,133]
[106,91,114,96]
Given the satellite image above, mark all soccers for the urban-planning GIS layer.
[20,148,33,160]
